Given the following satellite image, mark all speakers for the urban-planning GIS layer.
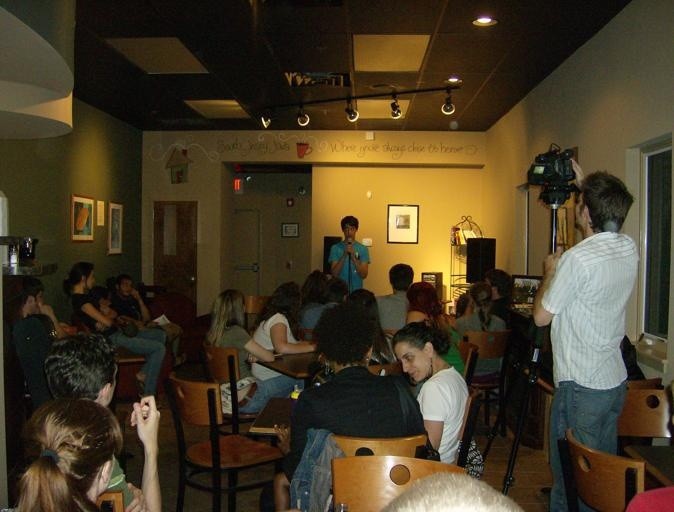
[466,238,496,283]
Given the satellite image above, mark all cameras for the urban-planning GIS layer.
[525,142,581,208]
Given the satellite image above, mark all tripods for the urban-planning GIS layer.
[483,208,556,496]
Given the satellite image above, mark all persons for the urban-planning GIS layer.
[1,261,515,512]
[328,216,369,293]
[528,160,641,512]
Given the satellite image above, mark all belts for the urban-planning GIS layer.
[238,383,257,407]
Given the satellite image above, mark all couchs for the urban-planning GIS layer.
[114,296,195,402]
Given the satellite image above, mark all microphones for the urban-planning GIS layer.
[347,238,353,254]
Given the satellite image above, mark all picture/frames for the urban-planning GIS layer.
[387,204,419,245]
[510,274,544,305]
[69,194,95,241]
[280,221,300,238]
[108,202,124,256]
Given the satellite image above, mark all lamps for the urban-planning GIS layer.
[261,85,455,129]
